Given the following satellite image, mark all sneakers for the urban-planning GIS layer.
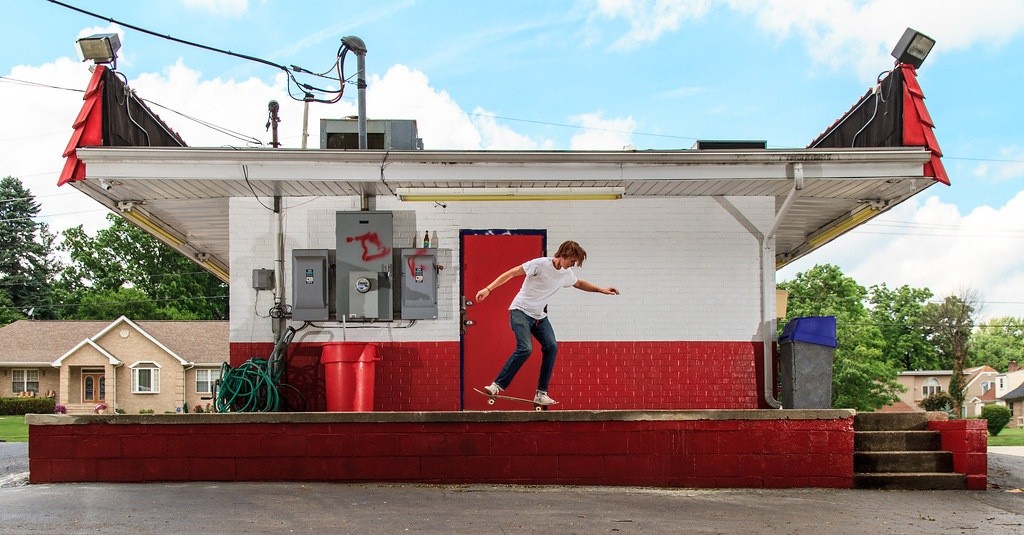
[534,394,556,404]
[484,384,499,395]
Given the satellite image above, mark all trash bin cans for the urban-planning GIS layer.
[778,316,837,409]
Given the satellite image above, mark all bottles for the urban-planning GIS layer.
[424,231,430,248]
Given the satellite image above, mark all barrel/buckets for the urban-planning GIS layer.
[320,342,379,412]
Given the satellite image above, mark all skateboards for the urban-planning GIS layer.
[472,387,560,411]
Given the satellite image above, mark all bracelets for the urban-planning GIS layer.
[486,287,491,293]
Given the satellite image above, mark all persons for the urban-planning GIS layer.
[476,240,621,405]
